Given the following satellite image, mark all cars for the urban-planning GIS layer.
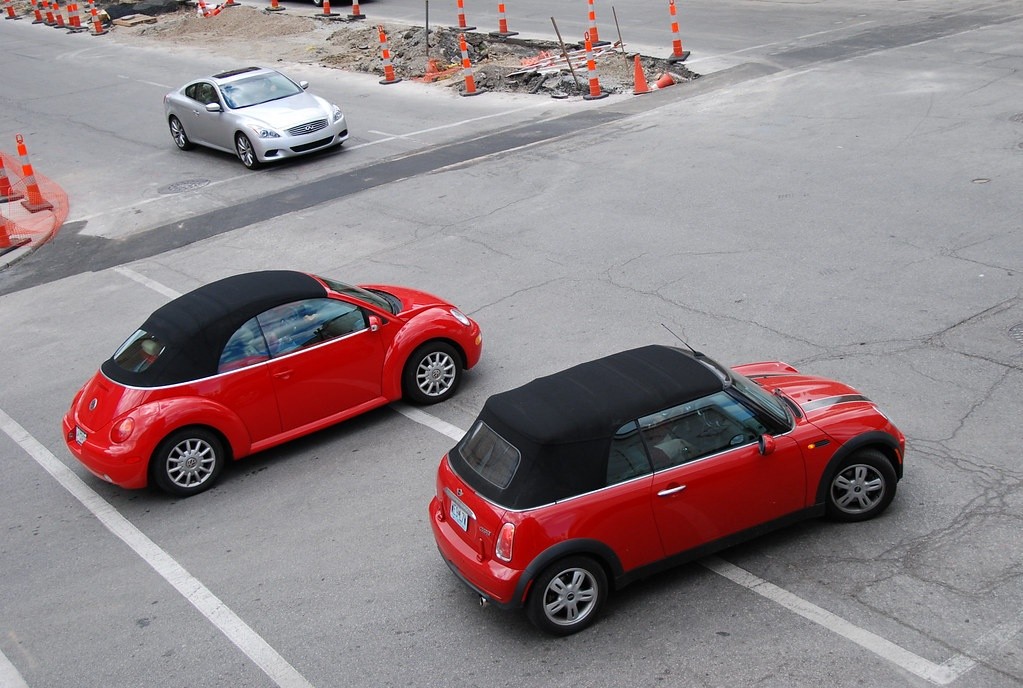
[61,269,485,501]
[427,323,907,641]
[163,66,350,171]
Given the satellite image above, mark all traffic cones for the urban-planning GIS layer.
[632,55,649,93]
